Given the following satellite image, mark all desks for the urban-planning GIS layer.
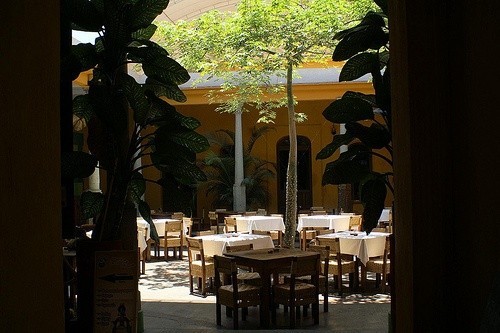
[150,211,174,219]
[191,232,275,296]
[315,230,392,294]
[297,214,352,241]
[222,247,321,330]
[137,219,188,264]
[215,210,245,234]
[223,215,286,249]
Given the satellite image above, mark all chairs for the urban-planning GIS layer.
[138,205,392,330]
